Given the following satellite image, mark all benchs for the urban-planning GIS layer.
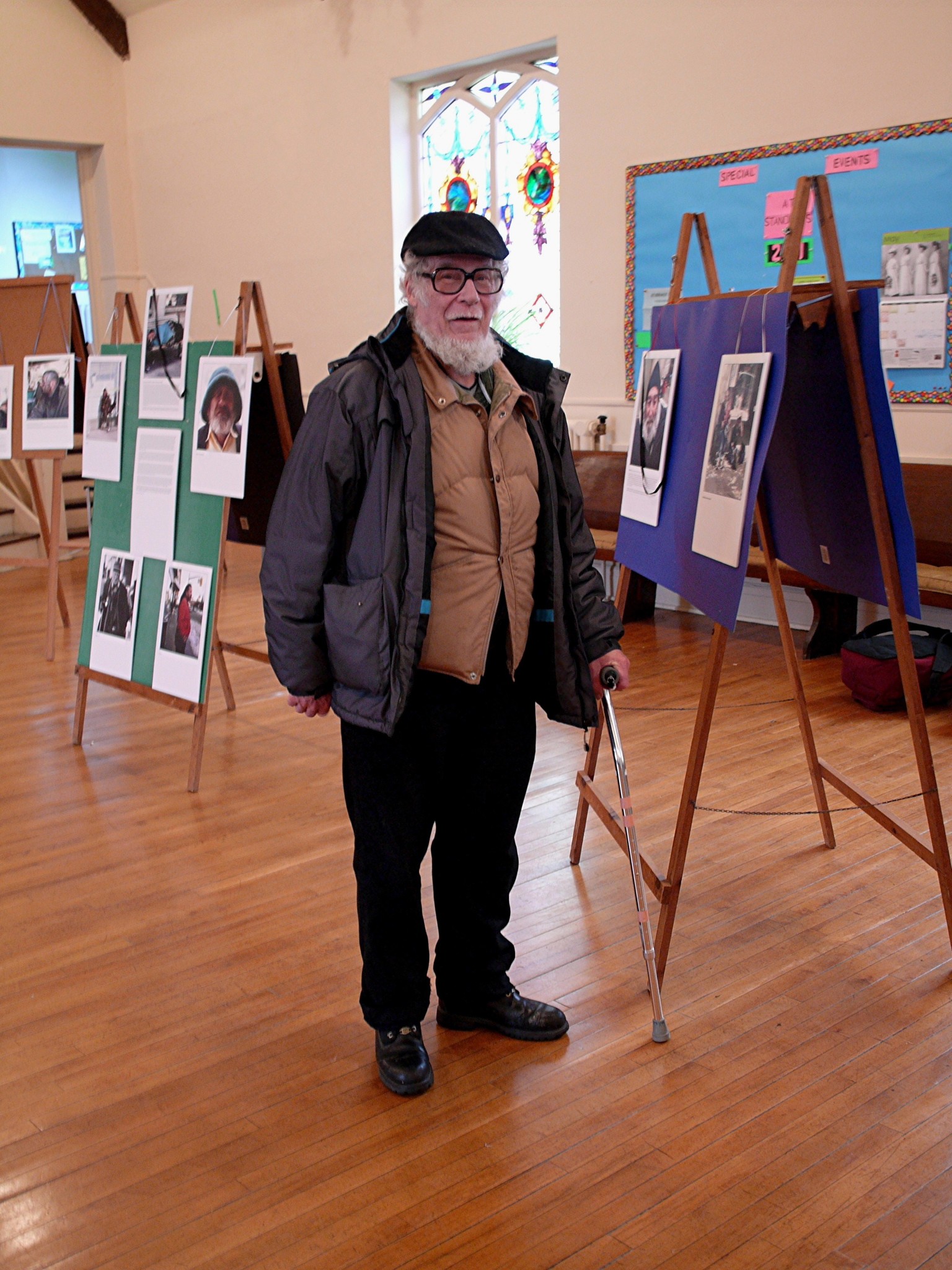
[569,449,952,659]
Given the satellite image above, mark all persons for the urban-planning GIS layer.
[175,584,193,653]
[98,561,130,638]
[197,367,241,453]
[258,211,631,1095]
[28,369,68,419]
[883,241,943,296]
[710,386,746,472]
[630,361,668,471]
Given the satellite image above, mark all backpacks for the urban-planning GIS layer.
[841,618,952,710]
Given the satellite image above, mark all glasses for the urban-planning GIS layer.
[414,266,505,294]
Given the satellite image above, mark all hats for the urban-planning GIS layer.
[201,366,242,423]
[401,210,509,262]
[646,361,660,394]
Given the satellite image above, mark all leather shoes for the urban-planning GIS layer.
[436,987,570,1041]
[375,1025,434,1094]
